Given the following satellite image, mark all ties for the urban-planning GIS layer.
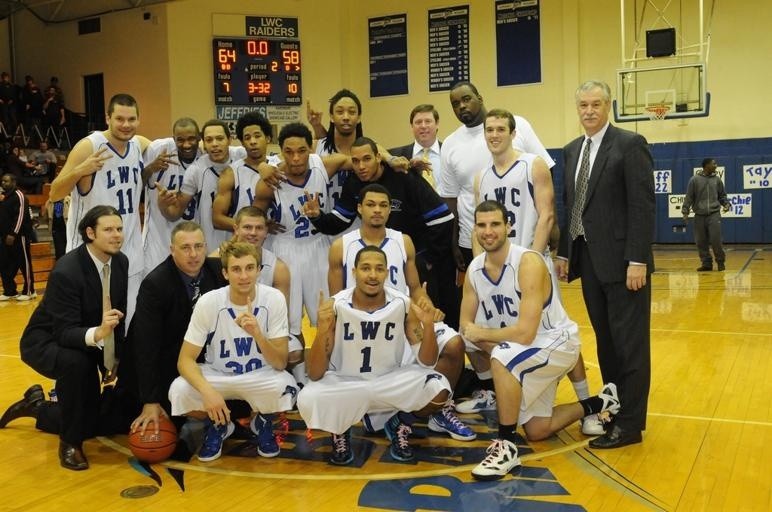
[421,148,435,187]
[100,265,116,371]
[189,278,205,310]
[568,140,593,240]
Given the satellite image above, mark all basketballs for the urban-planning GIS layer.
[129,416,176,462]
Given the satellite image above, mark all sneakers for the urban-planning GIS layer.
[250,410,281,458]
[428,393,477,441]
[16,291,37,301]
[384,410,415,462]
[471,438,521,480]
[238,409,288,435]
[197,418,235,461]
[456,389,497,414]
[581,414,610,436]
[1,294,18,301]
[328,427,355,466]
[598,382,621,415]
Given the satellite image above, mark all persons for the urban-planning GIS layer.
[458,199,622,482]
[49,79,609,443]
[555,77,657,450]
[680,157,734,272]
[1,204,130,470]
[295,245,455,466]
[1,70,72,302]
[167,232,302,464]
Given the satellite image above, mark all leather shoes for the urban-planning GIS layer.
[718,265,725,271]
[58,439,88,470]
[697,266,712,271]
[589,424,643,449]
[1,384,45,427]
